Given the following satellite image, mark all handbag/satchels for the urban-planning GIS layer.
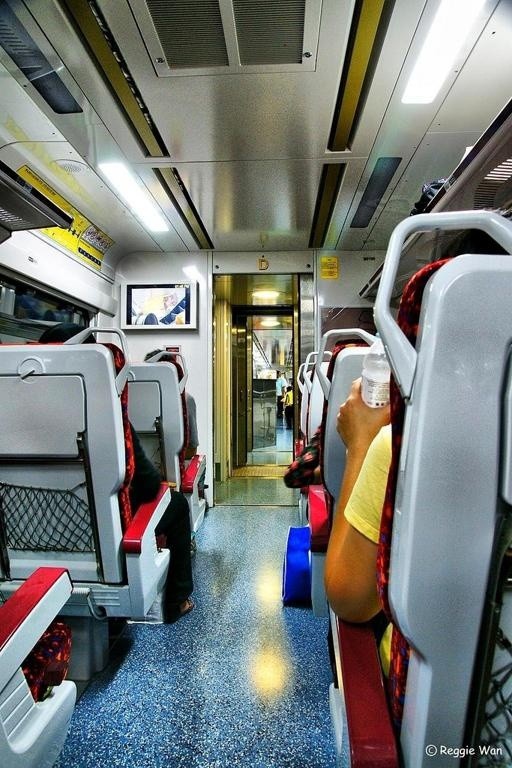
[409,178,448,216]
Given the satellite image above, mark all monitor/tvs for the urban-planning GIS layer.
[121,281,197,329]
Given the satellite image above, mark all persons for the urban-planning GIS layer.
[283,424,322,520]
[145,348,210,514]
[42,324,194,625]
[275,371,287,419]
[284,386,294,429]
[322,375,454,678]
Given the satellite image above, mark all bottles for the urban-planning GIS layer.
[360,332,391,407]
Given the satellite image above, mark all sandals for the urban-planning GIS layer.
[165,598,194,624]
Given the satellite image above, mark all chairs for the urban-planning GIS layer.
[297,209,510,766]
[0,325,207,762]
